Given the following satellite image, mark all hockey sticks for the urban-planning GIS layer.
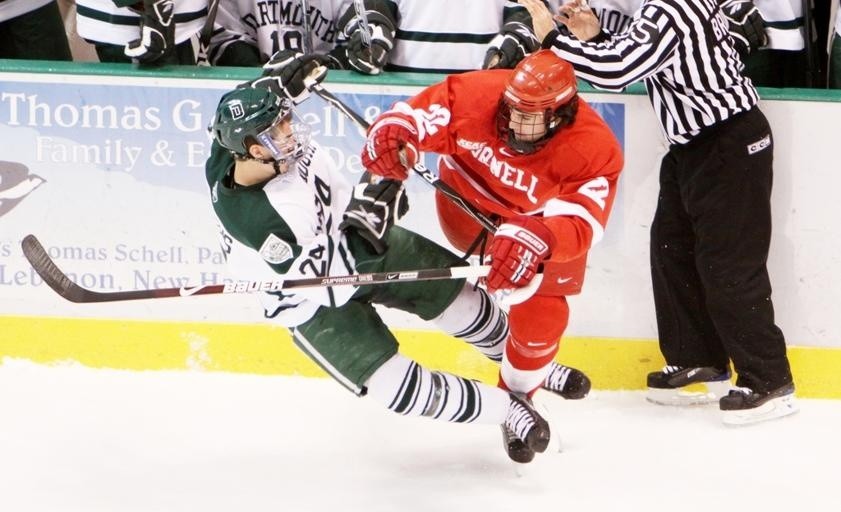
[21,235,495,302]
[309,82,544,308]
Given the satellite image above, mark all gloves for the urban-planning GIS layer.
[238,49,334,106]
[480,215,556,294]
[361,111,420,180]
[481,21,540,69]
[718,0,767,58]
[339,170,408,254]
[124,0,174,64]
[343,10,396,76]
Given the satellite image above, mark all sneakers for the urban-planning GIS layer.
[647,364,732,388]
[501,361,590,463]
[720,382,794,409]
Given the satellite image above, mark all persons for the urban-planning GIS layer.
[746,1,841,89]
[0,0,649,75]
[361,52,591,464]
[517,0,796,412]
[204,49,591,454]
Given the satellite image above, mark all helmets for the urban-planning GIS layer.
[496,50,578,154]
[208,87,312,162]
